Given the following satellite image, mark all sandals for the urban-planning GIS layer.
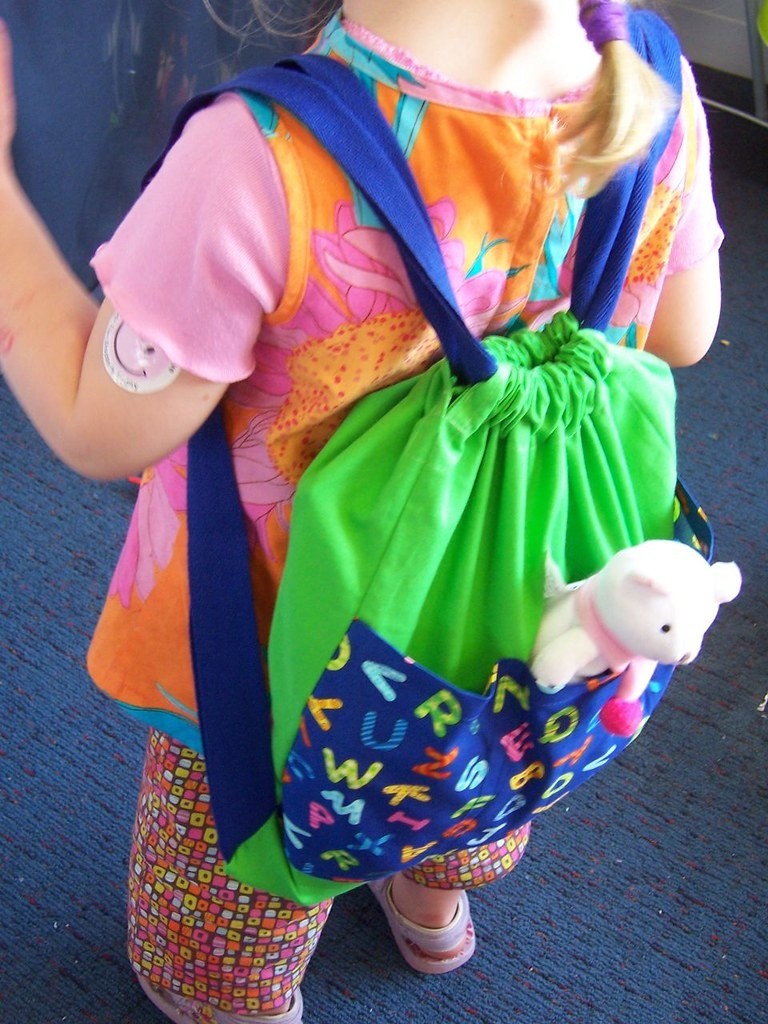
[137,974,304,1024]
[366,873,478,975]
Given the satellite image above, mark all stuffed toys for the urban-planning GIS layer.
[530,540,742,737]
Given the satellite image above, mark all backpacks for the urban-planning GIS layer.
[142,11,741,904]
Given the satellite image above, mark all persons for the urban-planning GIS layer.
[0,0,724,1024]
